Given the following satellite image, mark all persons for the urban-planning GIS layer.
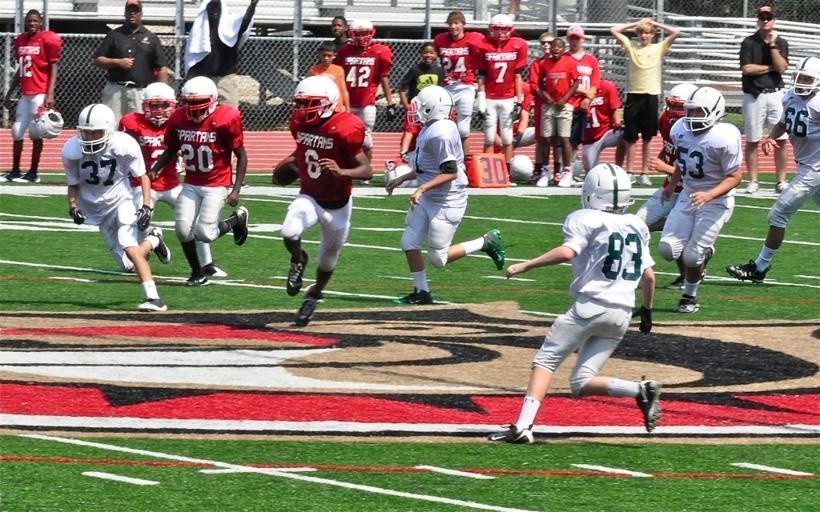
[608,17,680,188]
[535,37,581,190]
[636,83,715,283]
[396,41,446,165]
[330,14,358,76]
[739,5,790,196]
[724,51,820,284]
[60,102,172,314]
[306,40,351,114]
[184,0,258,79]
[528,28,563,186]
[385,85,506,307]
[658,87,745,315]
[94,1,169,120]
[333,17,397,186]
[562,22,600,190]
[432,11,480,157]
[147,75,250,289]
[486,158,660,444]
[117,81,229,281]
[468,14,529,189]
[573,81,628,174]
[476,75,536,158]
[1,8,62,185]
[271,73,374,327]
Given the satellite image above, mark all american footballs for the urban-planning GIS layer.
[274,156,299,185]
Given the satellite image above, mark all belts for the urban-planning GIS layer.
[760,86,780,93]
[115,80,144,88]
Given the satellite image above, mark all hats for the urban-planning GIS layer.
[566,24,585,41]
[125,0,143,9]
[758,5,775,15]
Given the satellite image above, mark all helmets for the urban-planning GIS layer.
[29,109,64,141]
[790,57,820,97]
[508,155,534,184]
[489,13,516,44]
[294,75,341,127]
[683,86,727,133]
[665,84,698,116]
[180,75,219,123]
[580,163,636,214]
[348,18,374,49]
[77,102,116,156]
[407,86,456,126]
[142,82,176,127]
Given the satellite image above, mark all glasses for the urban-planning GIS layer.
[758,15,771,20]
[126,8,140,13]
[539,40,553,45]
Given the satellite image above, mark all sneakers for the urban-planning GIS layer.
[393,287,435,307]
[482,229,506,272]
[0,173,21,183]
[625,171,639,185]
[186,270,209,287]
[231,205,249,246]
[295,283,319,327]
[537,166,552,186]
[745,180,759,194]
[636,379,662,431]
[665,277,688,290]
[286,249,309,295]
[203,262,230,278]
[775,181,789,192]
[528,169,541,185]
[639,173,652,187]
[553,171,561,183]
[558,168,574,189]
[677,292,700,313]
[14,172,41,184]
[489,425,535,448]
[137,298,169,312]
[150,226,170,266]
[725,259,772,285]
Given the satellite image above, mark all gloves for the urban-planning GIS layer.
[135,205,154,233]
[68,206,87,226]
[632,305,653,337]
